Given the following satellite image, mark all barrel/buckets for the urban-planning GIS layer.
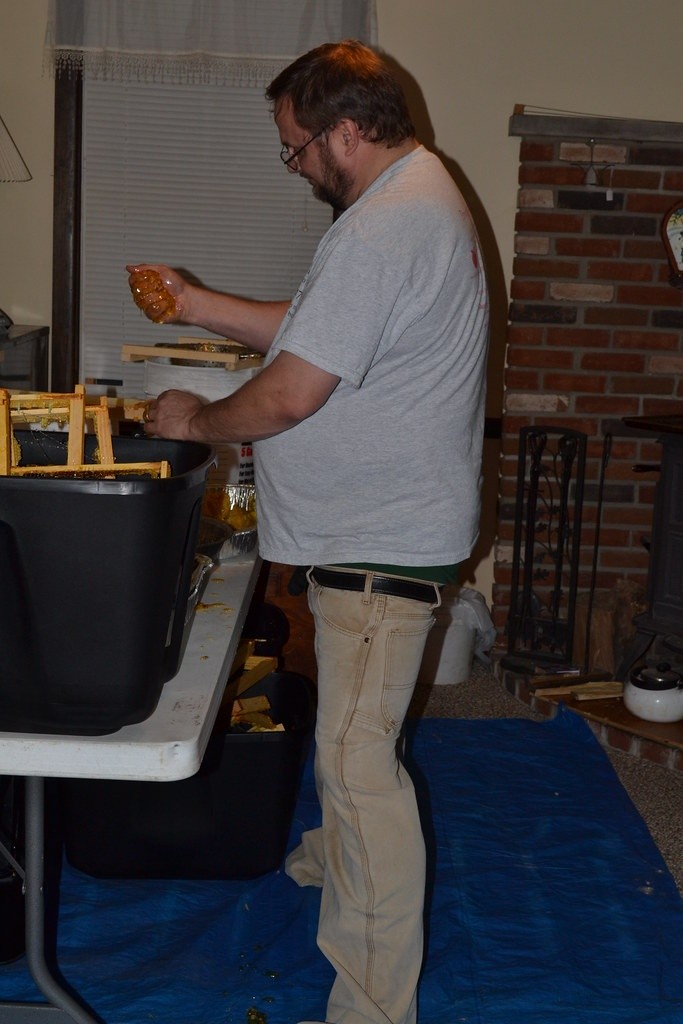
[419,585,485,686]
[141,345,266,487]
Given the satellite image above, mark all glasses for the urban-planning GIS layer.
[280,117,362,170]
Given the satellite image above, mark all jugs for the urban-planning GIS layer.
[623,662,683,724]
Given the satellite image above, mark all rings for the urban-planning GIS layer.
[145,403,150,421]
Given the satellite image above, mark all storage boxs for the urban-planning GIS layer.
[0,427,221,736]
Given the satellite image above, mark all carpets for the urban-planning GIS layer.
[0,698,683,1023]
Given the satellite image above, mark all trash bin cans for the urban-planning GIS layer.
[416,584,486,685]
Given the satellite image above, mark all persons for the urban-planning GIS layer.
[123,39,490,1024]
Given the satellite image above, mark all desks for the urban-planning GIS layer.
[0,550,266,1024]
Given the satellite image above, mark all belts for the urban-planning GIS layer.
[311,566,443,603]
